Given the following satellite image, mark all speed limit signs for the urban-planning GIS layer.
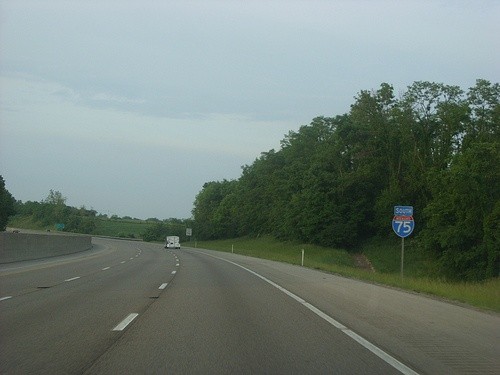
[392,215,415,238]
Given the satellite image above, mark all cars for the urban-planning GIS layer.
[164,236,180,249]
